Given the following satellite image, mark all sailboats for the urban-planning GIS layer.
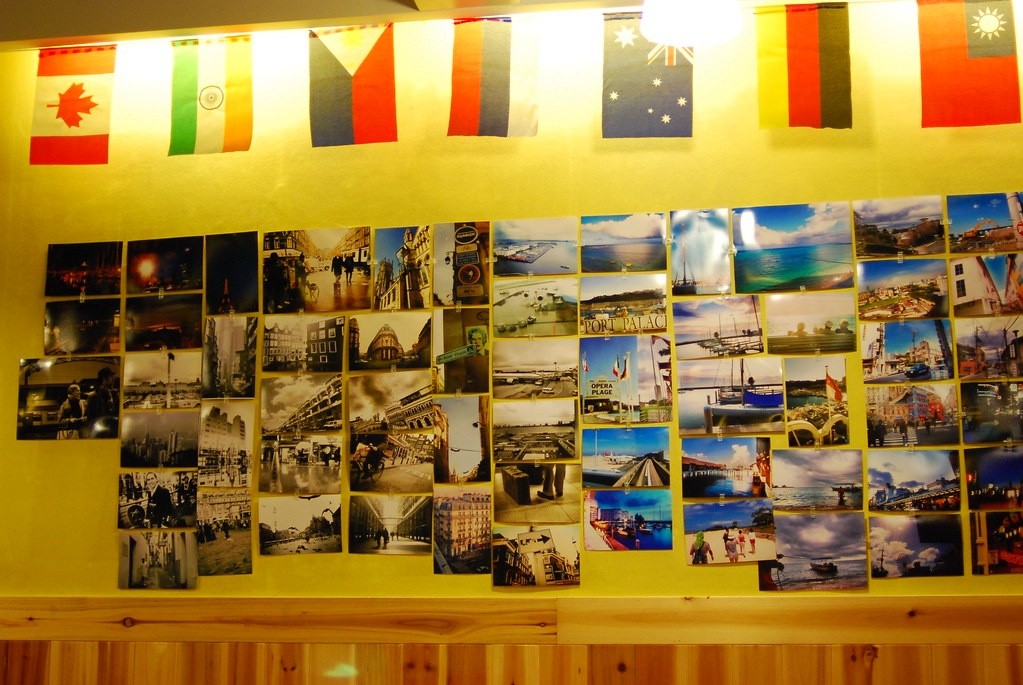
[704,358,784,422]
[672,223,697,297]
[696,295,761,353]
[872,546,888,578]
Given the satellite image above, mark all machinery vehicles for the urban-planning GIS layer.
[962,219,999,240]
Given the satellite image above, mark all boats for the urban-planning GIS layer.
[830,482,860,492]
[497,314,538,332]
[595,504,664,538]
[811,561,838,575]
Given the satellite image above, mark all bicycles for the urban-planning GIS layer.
[350,454,385,488]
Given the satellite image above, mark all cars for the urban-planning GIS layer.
[904,362,929,378]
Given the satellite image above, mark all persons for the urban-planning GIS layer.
[759,552,784,591]
[263,251,372,308]
[119,472,197,526]
[467,328,489,358]
[866,414,958,447]
[375,527,406,550]
[690,528,756,564]
[139,557,150,586]
[196,516,251,545]
[56,367,120,439]
[536,463,566,500]
[789,319,855,335]
[360,443,391,480]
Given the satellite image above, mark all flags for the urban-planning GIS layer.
[581,350,631,382]
[825,374,844,402]
